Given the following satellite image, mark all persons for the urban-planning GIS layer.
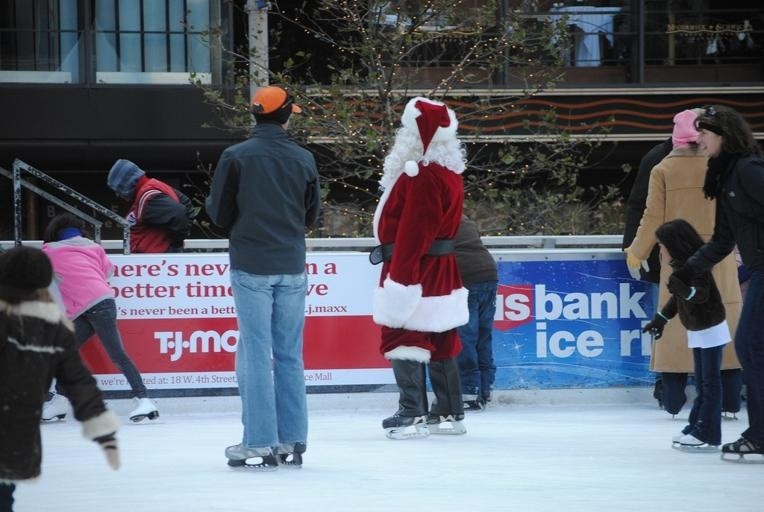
[204,86,322,460]
[368,95,470,429]
[428,211,498,405]
[625,107,743,421]
[641,217,733,454]
[696,24,727,65]
[37,213,160,421]
[622,138,672,408]
[665,106,764,462]
[106,158,192,253]
[729,16,758,54]
[0,244,120,511]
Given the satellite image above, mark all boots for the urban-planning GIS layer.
[43,397,71,419]
[129,397,160,419]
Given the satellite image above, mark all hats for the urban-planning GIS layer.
[401,97,458,177]
[109,159,145,195]
[2,248,53,307]
[251,88,302,123]
[671,109,701,146]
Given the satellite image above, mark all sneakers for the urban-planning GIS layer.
[226,437,304,459]
[424,412,466,424]
[460,397,484,408]
[673,429,702,446]
[382,416,427,427]
[722,437,761,455]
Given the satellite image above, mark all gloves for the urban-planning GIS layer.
[98,435,120,469]
[642,313,668,340]
[622,247,649,282]
[666,257,700,300]
